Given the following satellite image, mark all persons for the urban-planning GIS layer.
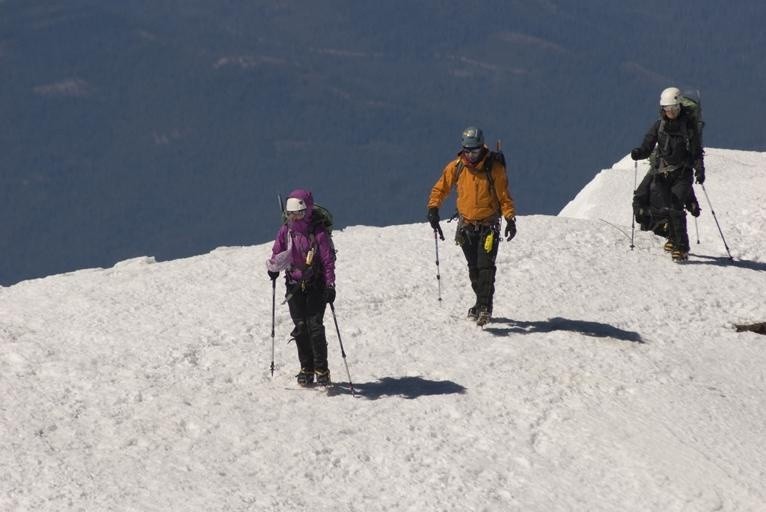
[427,126,517,325]
[630,86,704,263]
[268,190,335,387]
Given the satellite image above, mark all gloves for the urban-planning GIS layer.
[427,207,440,228]
[505,219,516,241]
[268,270,279,279]
[632,148,641,160]
[323,288,335,302]
[696,168,704,184]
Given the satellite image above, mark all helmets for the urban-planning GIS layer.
[659,88,680,105]
[462,128,483,148]
[286,198,307,212]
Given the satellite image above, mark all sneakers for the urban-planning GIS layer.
[315,368,330,382]
[664,238,674,251]
[673,249,688,258]
[298,367,314,383]
[468,303,491,325]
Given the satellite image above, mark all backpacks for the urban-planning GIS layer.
[309,204,333,241]
[677,97,699,125]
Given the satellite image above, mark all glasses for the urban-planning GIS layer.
[464,148,482,153]
